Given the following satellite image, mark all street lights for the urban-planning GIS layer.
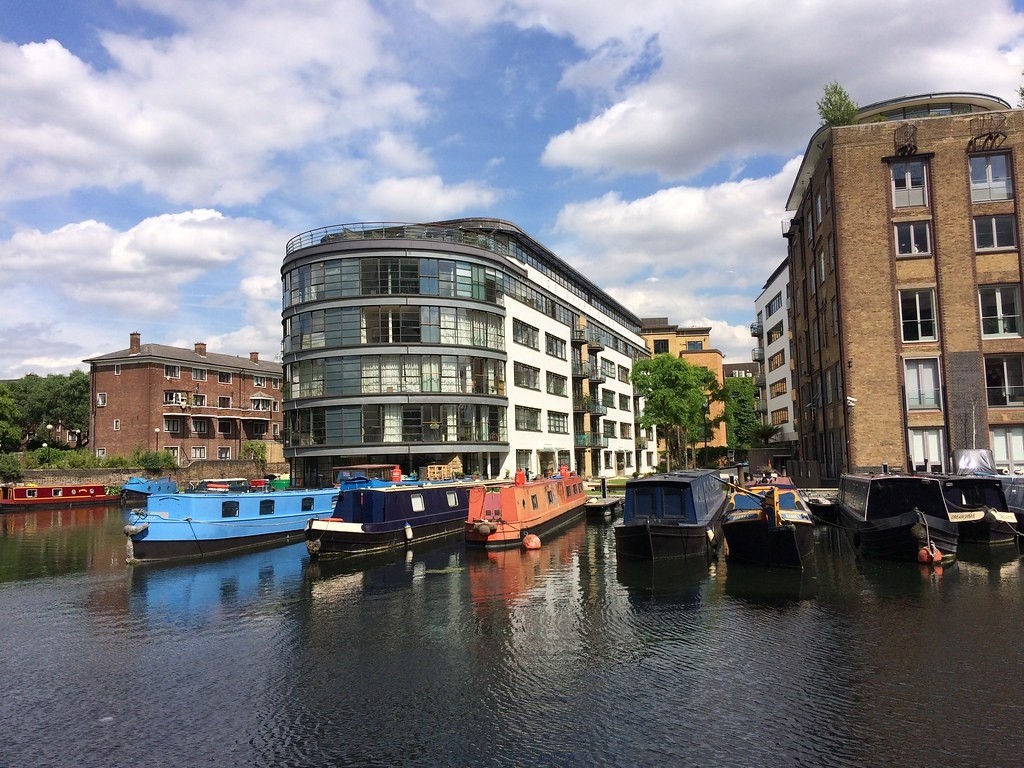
[46,421,53,465]
[154,428,160,451]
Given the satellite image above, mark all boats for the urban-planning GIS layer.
[950,448,1024,534]
[721,478,816,565]
[0,483,122,509]
[464,466,587,545]
[250,473,280,493]
[121,473,179,508]
[802,495,832,507]
[837,473,923,538]
[124,464,483,560]
[614,468,734,566]
[304,477,515,557]
[184,478,250,495]
[913,471,1019,556]
